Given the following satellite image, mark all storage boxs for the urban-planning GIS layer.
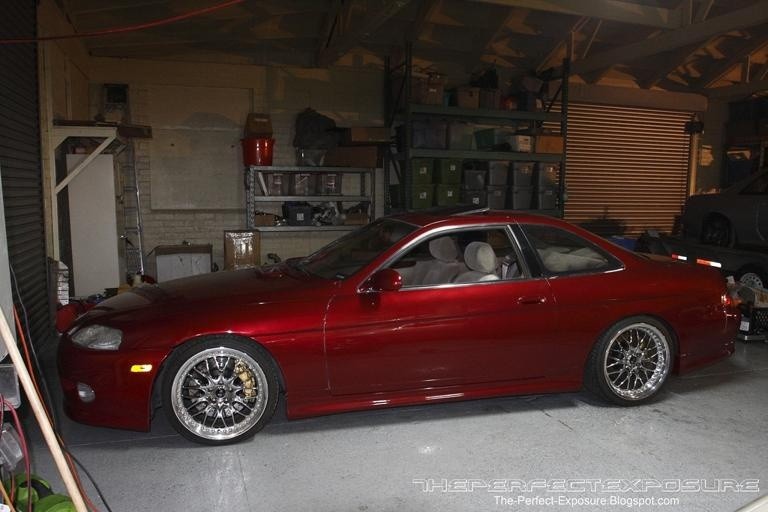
[224,230,260,272]
[326,126,382,168]
[386,66,558,211]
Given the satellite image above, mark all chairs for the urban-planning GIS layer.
[411,235,598,285]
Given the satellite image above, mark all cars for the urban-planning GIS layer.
[683,167,768,251]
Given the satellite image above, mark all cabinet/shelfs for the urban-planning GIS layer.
[65,153,127,300]
[244,165,376,232]
[382,22,570,223]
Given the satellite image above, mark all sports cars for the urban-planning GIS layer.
[53,206,742,448]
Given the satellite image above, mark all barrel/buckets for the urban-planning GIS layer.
[240,136,275,166]
